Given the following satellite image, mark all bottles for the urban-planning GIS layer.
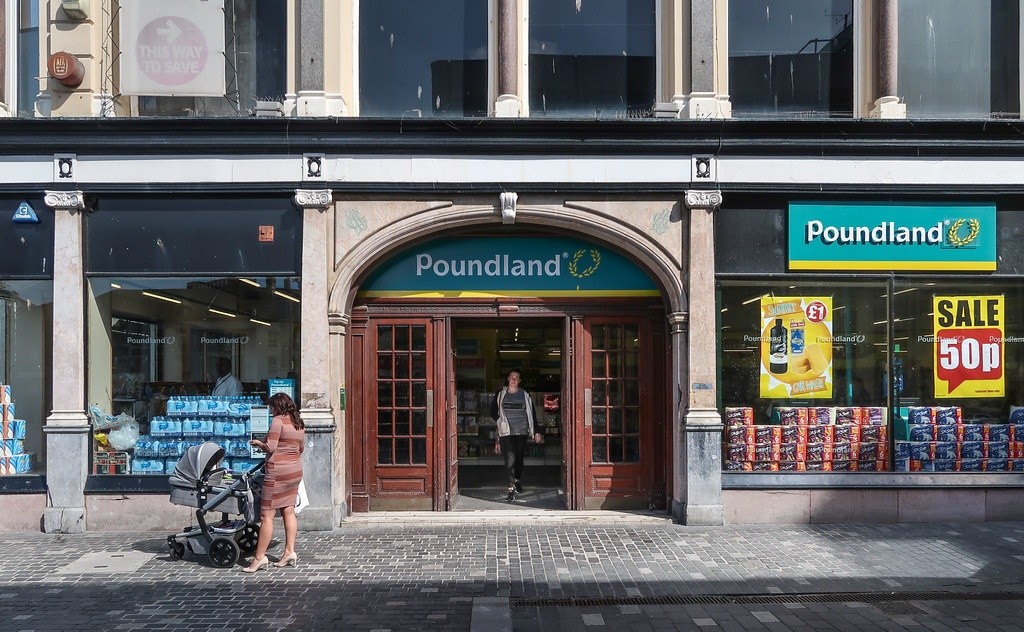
[132,394,273,475]
[770,319,788,374]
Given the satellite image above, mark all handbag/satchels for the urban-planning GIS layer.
[294,477,309,513]
[243,477,261,524]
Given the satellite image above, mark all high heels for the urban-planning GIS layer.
[243,556,269,572]
[274,552,297,567]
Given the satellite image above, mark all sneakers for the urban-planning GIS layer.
[505,490,515,502]
[514,480,521,494]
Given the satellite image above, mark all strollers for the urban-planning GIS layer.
[167,441,265,568]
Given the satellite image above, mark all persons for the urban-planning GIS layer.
[242,393,305,572]
[491,369,541,501]
[212,357,242,396]
